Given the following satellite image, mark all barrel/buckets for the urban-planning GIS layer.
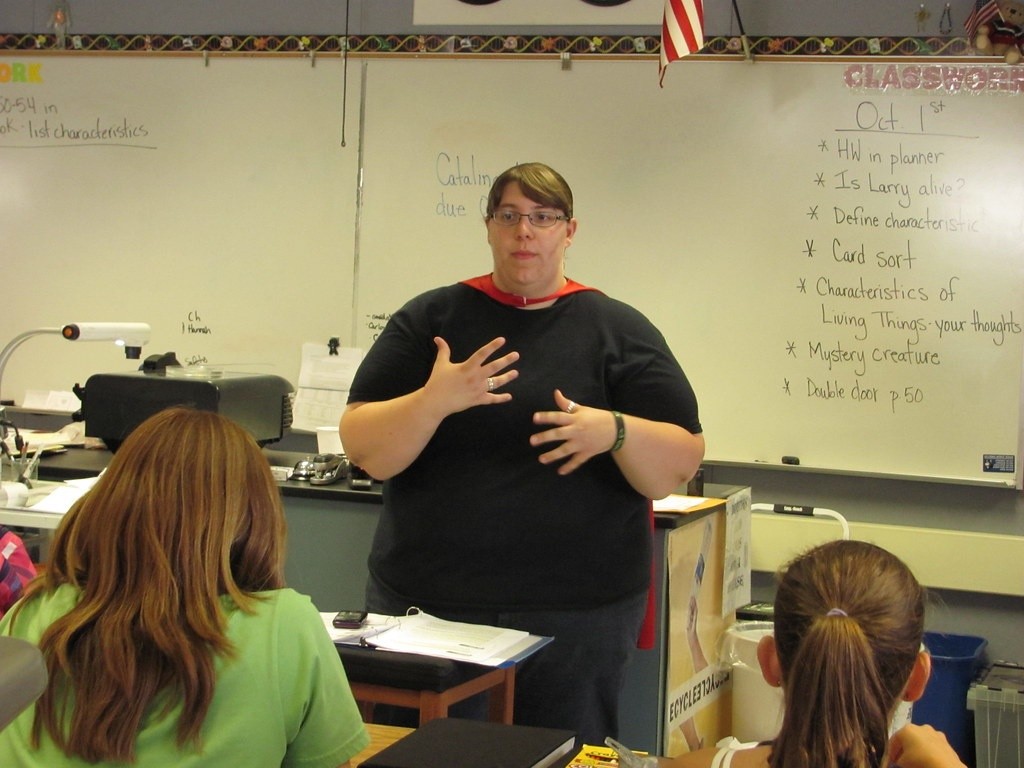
[916,629,990,768]
[888,641,926,738]
[728,620,786,743]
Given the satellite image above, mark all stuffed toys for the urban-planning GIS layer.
[974,0,1024,66]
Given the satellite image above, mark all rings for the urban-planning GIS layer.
[486,377,494,392]
[565,400,575,414]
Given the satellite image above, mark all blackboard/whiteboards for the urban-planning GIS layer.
[0,45,1024,489]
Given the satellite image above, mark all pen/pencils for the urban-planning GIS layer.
[0,434,46,480]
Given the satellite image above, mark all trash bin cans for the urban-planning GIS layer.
[728,619,788,744]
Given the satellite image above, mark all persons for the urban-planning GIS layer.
[677,715,704,753]
[0,404,372,768]
[337,161,706,752]
[685,596,709,674]
[664,539,970,768]
[0,526,37,622]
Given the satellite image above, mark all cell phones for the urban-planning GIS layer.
[333,610,368,629]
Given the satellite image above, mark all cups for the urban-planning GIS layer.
[11,457,40,490]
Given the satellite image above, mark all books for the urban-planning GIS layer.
[317,607,555,670]
[564,743,648,768]
[355,716,581,768]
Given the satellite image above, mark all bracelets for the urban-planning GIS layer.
[607,410,625,453]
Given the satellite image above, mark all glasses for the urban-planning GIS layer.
[489,210,568,227]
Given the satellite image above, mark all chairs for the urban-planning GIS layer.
[749,503,851,541]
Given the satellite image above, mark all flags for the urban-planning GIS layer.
[962,0,1001,49]
[658,0,705,89]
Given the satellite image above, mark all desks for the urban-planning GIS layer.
[317,607,519,732]
[0,476,89,564]
[343,713,646,766]
[266,452,751,756]
